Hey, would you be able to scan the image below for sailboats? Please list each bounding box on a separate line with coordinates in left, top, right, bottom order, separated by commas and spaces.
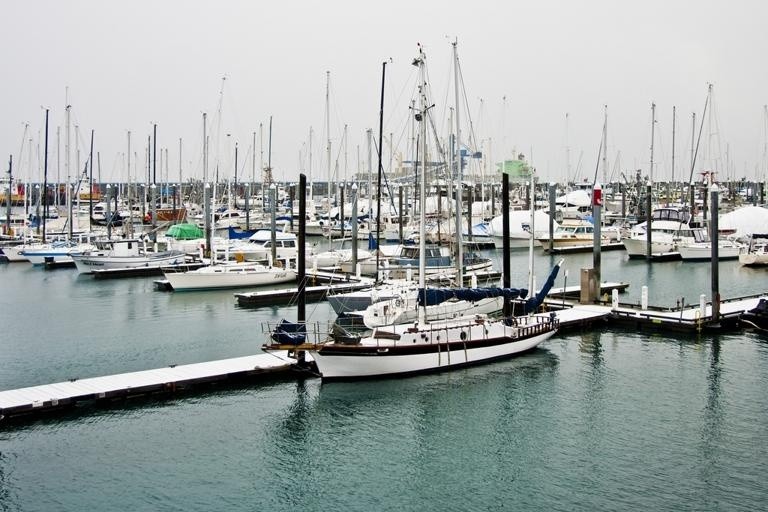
474, 83, 766, 272
0, 70, 393, 295
260, 35, 562, 398
